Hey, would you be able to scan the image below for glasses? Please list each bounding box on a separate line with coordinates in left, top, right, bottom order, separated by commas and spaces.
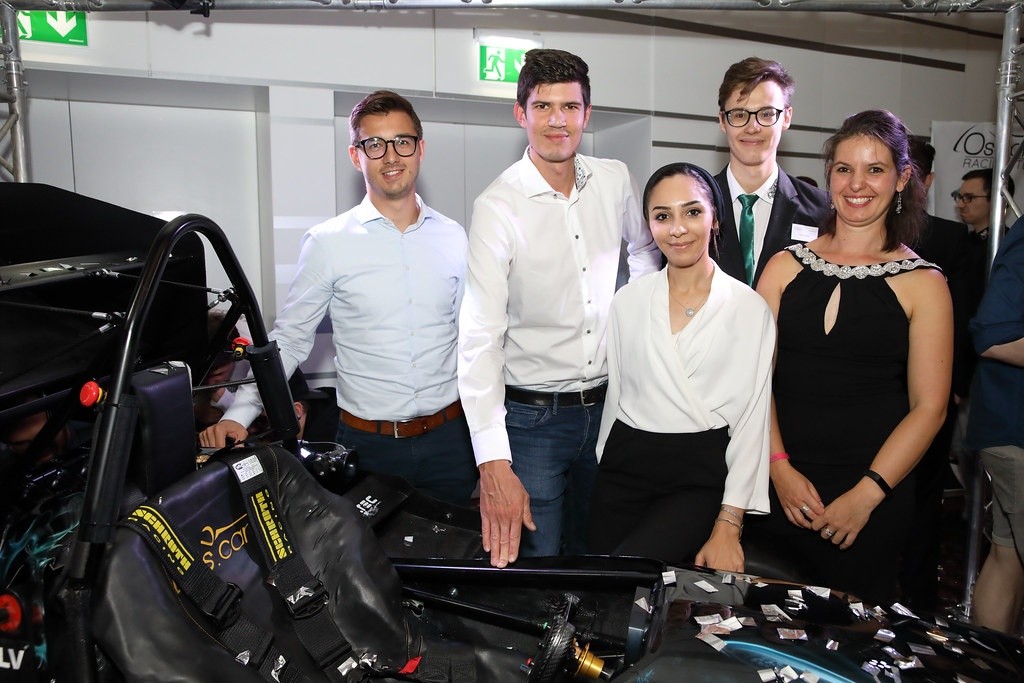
723, 107, 786, 128
951, 188, 989, 204
355, 135, 419, 159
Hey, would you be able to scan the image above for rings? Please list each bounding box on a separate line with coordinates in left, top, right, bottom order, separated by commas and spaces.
823, 526, 833, 537
801, 505, 809, 514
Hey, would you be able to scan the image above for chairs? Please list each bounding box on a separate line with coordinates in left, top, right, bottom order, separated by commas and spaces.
95, 448, 533, 683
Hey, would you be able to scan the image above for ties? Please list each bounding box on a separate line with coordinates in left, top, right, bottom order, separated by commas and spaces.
737, 194, 760, 287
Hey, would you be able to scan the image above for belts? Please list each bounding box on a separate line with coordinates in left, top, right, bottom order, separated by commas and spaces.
339, 399, 463, 439
505, 380, 608, 407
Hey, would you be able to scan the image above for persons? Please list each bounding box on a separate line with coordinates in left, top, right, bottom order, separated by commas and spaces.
190, 310, 338, 453
197, 48, 1024, 682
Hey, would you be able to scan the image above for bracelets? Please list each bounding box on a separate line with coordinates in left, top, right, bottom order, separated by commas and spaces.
770, 452, 790, 463
720, 506, 742, 522
714, 517, 743, 538
863, 469, 893, 497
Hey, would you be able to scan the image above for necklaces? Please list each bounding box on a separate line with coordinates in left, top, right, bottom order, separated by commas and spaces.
670, 293, 711, 317
